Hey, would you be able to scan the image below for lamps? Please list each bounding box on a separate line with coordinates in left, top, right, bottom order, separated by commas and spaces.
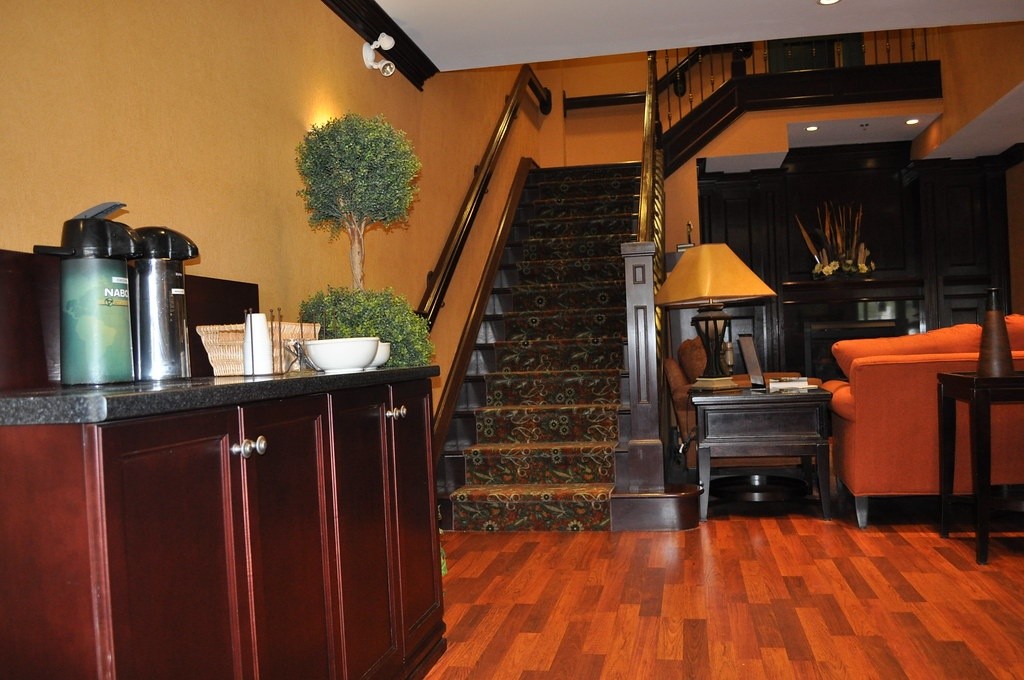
362, 32, 396, 77
656, 243, 777, 394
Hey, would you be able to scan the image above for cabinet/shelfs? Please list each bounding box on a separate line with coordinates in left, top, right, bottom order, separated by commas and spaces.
0, 364, 449, 680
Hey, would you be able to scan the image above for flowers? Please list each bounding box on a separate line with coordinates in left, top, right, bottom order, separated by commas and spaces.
812, 243, 876, 276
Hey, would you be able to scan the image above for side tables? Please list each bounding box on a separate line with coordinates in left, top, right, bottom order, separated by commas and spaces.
687, 387, 833, 521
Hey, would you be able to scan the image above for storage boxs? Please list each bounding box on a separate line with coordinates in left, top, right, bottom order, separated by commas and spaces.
196, 322, 321, 376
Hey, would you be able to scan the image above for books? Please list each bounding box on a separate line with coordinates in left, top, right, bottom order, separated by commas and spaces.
769, 377, 818, 393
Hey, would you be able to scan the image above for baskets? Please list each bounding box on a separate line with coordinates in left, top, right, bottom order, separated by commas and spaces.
196, 320, 322, 376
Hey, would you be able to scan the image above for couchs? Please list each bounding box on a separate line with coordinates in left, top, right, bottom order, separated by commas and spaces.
665, 336, 822, 496
820, 313, 1024, 529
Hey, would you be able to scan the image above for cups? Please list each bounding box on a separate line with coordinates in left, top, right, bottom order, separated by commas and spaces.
242, 312, 273, 375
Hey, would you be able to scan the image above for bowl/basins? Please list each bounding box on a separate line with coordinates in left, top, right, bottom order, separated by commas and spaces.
304, 337, 380, 376
364, 342, 390, 371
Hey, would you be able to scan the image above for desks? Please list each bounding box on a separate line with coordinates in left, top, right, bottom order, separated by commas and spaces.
930, 370, 1024, 561
